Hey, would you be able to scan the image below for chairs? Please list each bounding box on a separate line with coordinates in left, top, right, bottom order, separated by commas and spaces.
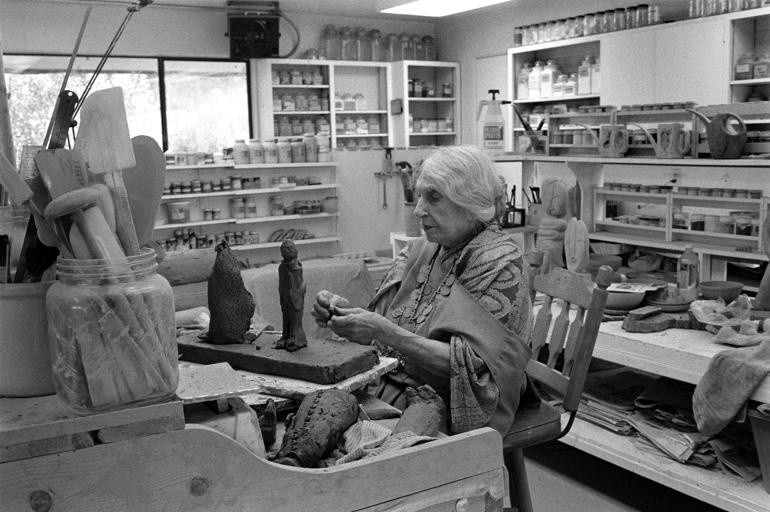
500, 248, 614, 511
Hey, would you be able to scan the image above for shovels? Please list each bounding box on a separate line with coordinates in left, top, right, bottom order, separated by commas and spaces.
35, 148, 89, 200
80, 87, 136, 258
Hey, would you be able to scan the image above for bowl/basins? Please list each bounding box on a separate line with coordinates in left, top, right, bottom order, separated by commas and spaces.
647, 288, 695, 313
701, 279, 743, 302
627, 277, 677, 292
594, 281, 645, 309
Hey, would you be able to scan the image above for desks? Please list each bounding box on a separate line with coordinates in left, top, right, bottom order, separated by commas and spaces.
0, 396, 506, 512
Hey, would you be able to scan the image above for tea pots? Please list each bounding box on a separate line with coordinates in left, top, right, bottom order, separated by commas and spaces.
629, 122, 689, 159
577, 121, 630, 158
685, 106, 747, 159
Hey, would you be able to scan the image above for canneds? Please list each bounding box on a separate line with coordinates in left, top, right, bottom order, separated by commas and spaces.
157, 238, 177, 252
198, 231, 260, 248
407, 76, 453, 98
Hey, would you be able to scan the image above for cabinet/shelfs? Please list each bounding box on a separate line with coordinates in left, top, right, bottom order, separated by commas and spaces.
153, 161, 340, 263
251, 57, 462, 151
504, 7, 770, 286
492, 100, 770, 511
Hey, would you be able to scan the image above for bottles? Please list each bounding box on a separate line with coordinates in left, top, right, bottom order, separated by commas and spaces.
386, 32, 401, 62
222, 178, 231, 190
231, 198, 245, 218
517, 63, 528, 99
593, 2, 656, 34
231, 175, 241, 189
540, 59, 561, 99
274, 116, 329, 136
555, 14, 594, 40
172, 181, 181, 193
410, 110, 453, 133
211, 180, 221, 191
291, 137, 306, 163
357, 25, 372, 61
232, 139, 250, 165
317, 135, 331, 163
522, 20, 555, 44
277, 137, 293, 163
590, 54, 601, 93
246, 197, 257, 218
182, 181, 191, 193
46, 247, 180, 413
272, 68, 324, 84
410, 33, 426, 60
370, 28, 385, 62
273, 91, 329, 110
249, 138, 265, 164
513, 26, 522, 46
577, 55, 592, 95
163, 183, 171, 194
734, 50, 754, 81
202, 180, 212, 192
687, 0, 769, 18
754, 47, 770, 80
302, 133, 320, 162
319, 24, 340, 60
193, 179, 201, 192
565, 72, 577, 97
335, 92, 367, 110
528, 61, 542, 99
398, 32, 414, 60
335, 115, 381, 135
175, 229, 197, 249
262, 138, 279, 164
553, 74, 567, 98
423, 35, 440, 61
271, 195, 284, 216
341, 25, 356, 60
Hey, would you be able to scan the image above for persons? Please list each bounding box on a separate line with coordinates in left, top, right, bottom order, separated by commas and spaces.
311, 144, 544, 439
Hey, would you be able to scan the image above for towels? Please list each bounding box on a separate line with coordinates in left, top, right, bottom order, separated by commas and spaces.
692, 327, 770, 437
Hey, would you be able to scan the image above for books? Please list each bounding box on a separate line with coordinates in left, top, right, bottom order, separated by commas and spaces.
530, 361, 761, 484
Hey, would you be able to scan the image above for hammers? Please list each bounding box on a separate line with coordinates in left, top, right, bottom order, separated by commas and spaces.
43, 187, 108, 258
